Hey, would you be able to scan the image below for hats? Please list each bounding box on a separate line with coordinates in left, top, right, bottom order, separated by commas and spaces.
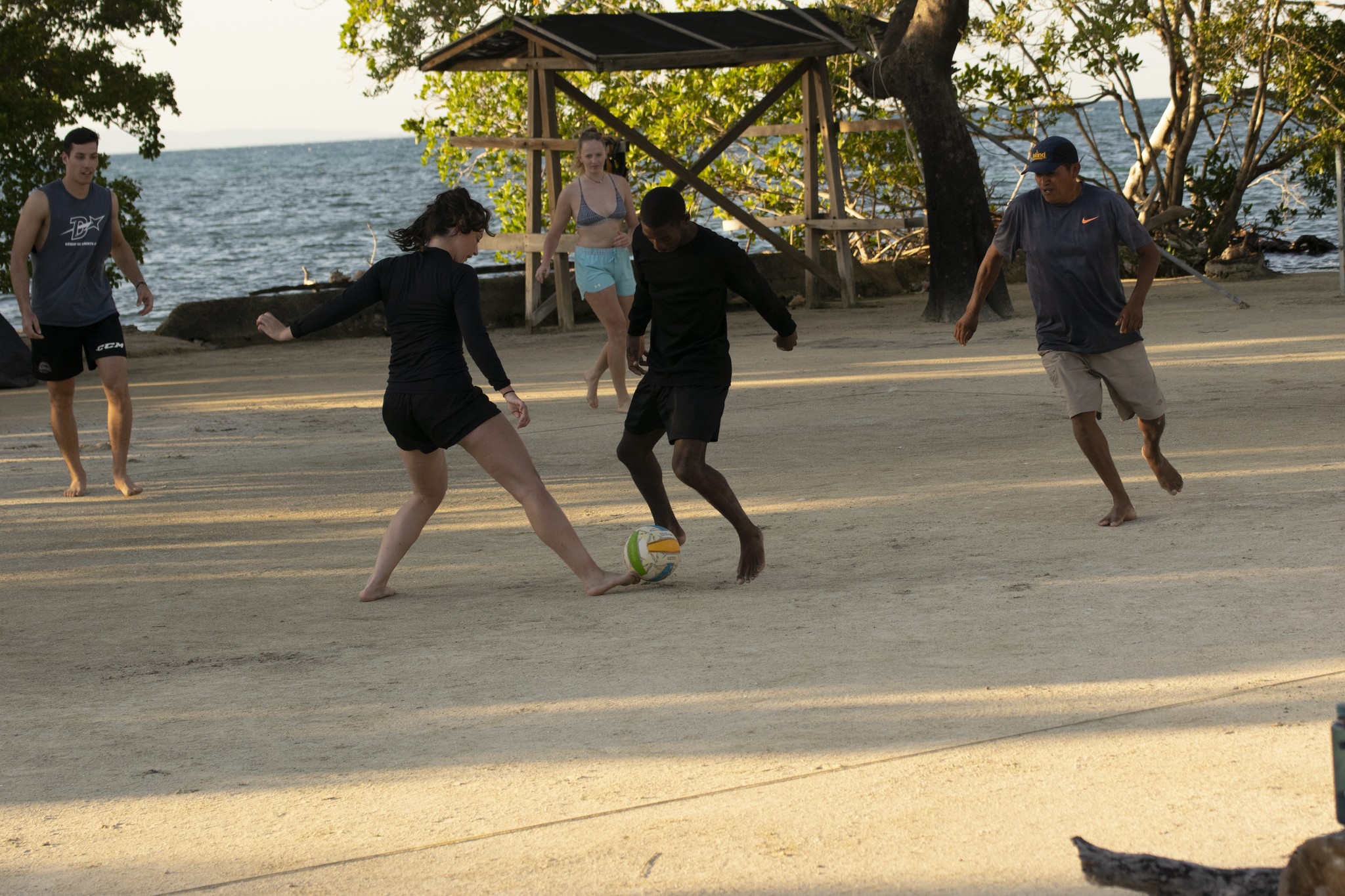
1020, 136, 1079, 175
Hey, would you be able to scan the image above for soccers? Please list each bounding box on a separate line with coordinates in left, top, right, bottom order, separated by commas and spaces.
623, 525, 681, 582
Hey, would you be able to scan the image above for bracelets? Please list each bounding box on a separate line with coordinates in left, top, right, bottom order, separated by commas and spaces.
503, 390, 515, 398
135, 282, 147, 291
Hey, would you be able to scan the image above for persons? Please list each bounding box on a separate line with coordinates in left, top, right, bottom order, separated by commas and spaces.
535, 128, 640, 413
10, 128, 153, 498
951, 136, 1186, 526
618, 187, 798, 584
256, 183, 641, 601
